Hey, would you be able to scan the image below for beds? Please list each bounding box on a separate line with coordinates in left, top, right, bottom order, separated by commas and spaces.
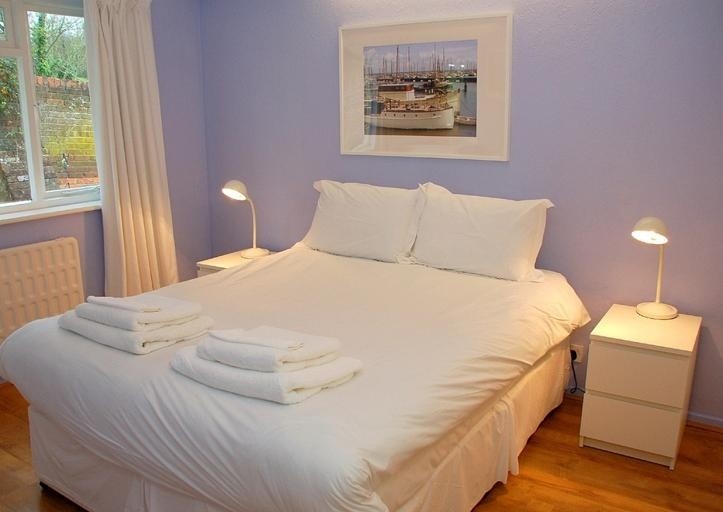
28, 243, 572, 511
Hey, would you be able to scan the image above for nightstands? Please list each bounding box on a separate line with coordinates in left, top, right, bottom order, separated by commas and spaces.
197, 251, 276, 278
578, 304, 702, 470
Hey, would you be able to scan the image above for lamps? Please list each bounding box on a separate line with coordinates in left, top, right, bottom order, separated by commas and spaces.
631, 217, 678, 319
221, 180, 269, 258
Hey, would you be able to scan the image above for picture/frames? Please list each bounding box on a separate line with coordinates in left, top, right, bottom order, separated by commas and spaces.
338, 11, 512, 163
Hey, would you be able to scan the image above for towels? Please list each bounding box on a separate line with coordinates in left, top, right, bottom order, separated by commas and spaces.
197, 326, 336, 372
171, 344, 360, 404
57, 310, 212, 355
76, 295, 201, 331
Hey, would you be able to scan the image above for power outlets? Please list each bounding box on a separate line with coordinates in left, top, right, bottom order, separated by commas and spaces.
570, 344, 582, 363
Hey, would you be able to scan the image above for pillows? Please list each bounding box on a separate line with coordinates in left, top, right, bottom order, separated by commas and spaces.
408, 181, 554, 282
300, 180, 425, 264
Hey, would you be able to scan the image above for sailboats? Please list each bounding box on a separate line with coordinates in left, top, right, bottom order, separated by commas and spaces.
364, 45, 476, 130
